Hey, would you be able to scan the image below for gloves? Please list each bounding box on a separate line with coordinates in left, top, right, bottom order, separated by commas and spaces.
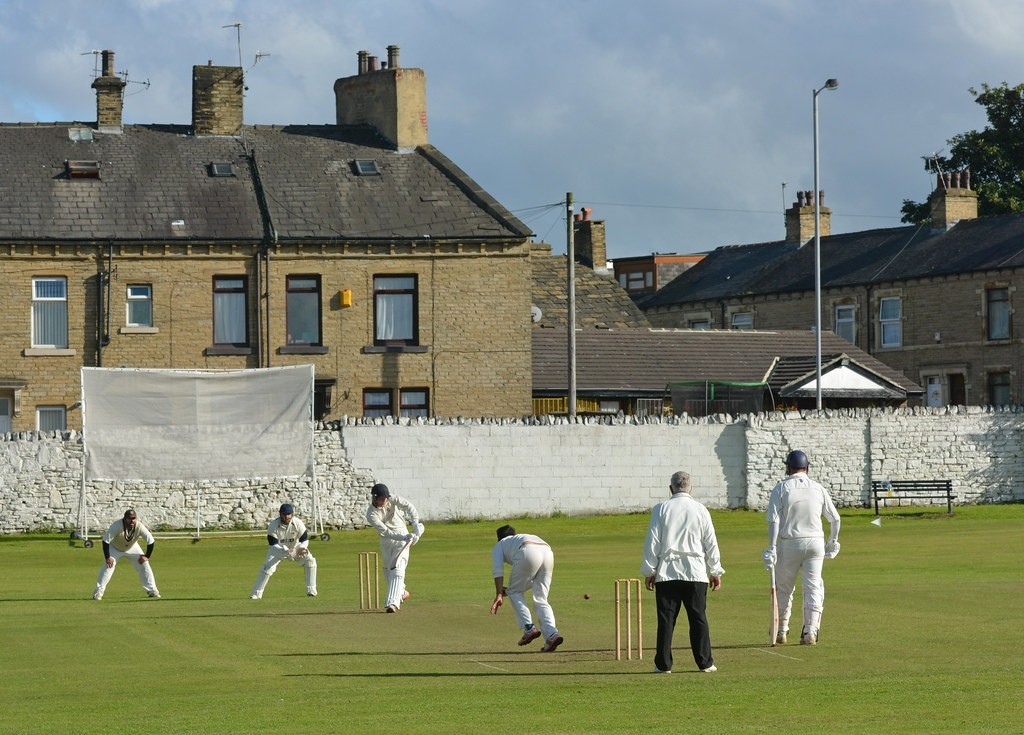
825, 542, 840, 559
411, 521, 425, 538
404, 533, 420, 545
760, 547, 777, 571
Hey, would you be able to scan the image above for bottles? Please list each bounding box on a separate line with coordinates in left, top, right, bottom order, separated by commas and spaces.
888, 482, 892, 496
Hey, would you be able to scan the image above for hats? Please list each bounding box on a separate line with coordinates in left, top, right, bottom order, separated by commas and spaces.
371, 484, 391, 497
281, 504, 293, 514
124, 510, 137, 518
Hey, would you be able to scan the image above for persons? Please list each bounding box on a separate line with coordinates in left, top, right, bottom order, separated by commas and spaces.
490, 525, 563, 653
644, 471, 725, 673
93, 510, 162, 601
367, 484, 421, 614
764, 451, 841, 645
249, 504, 318, 600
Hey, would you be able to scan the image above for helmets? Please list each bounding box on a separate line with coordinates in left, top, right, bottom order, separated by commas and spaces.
785, 450, 810, 476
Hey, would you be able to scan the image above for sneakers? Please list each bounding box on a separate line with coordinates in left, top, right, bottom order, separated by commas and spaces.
518, 624, 540, 646
540, 633, 564, 651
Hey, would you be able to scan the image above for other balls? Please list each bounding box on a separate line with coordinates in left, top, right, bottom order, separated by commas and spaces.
584, 593, 590, 600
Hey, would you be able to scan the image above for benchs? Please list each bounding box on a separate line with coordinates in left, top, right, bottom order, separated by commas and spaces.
870, 475, 958, 516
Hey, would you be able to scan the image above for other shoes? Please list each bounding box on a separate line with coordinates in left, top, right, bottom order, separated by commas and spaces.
250, 593, 258, 599
654, 666, 672, 674
400, 590, 410, 604
149, 593, 162, 597
700, 664, 717, 672
92, 595, 102, 600
776, 630, 787, 644
386, 606, 396, 613
800, 632, 817, 646
306, 588, 317, 596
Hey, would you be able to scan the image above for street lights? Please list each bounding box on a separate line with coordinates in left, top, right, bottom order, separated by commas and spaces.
811, 78, 838, 409
